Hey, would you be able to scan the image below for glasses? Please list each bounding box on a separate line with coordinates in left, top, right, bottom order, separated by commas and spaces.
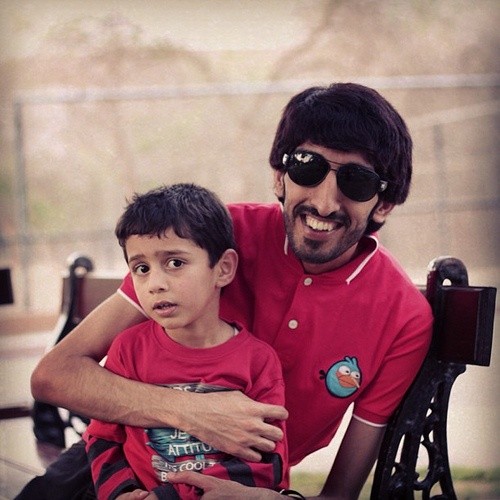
282, 150, 388, 202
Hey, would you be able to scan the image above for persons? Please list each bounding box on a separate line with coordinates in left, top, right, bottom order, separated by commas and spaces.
12, 82, 434, 499
82, 183, 291, 499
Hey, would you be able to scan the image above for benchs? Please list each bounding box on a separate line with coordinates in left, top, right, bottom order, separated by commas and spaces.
0, 256, 497, 500
